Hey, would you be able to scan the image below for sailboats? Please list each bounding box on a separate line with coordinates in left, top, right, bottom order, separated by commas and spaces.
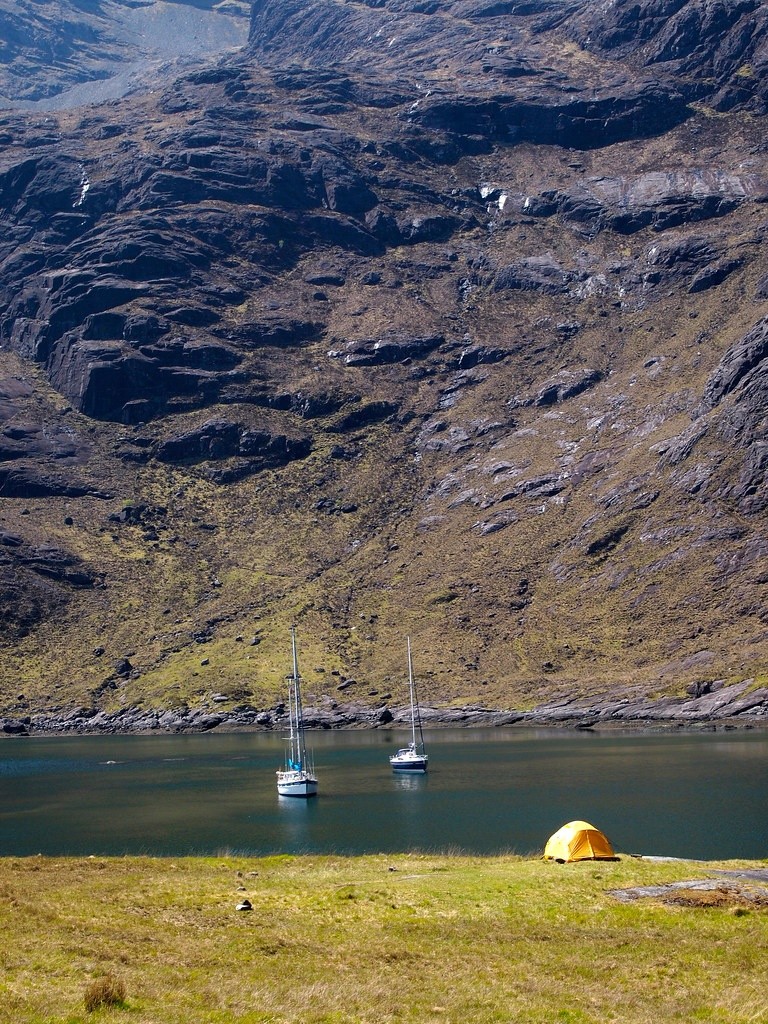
388, 635, 429, 774
274, 626, 319, 798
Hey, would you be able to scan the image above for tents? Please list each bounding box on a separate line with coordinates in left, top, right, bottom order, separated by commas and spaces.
542, 820, 621, 864
